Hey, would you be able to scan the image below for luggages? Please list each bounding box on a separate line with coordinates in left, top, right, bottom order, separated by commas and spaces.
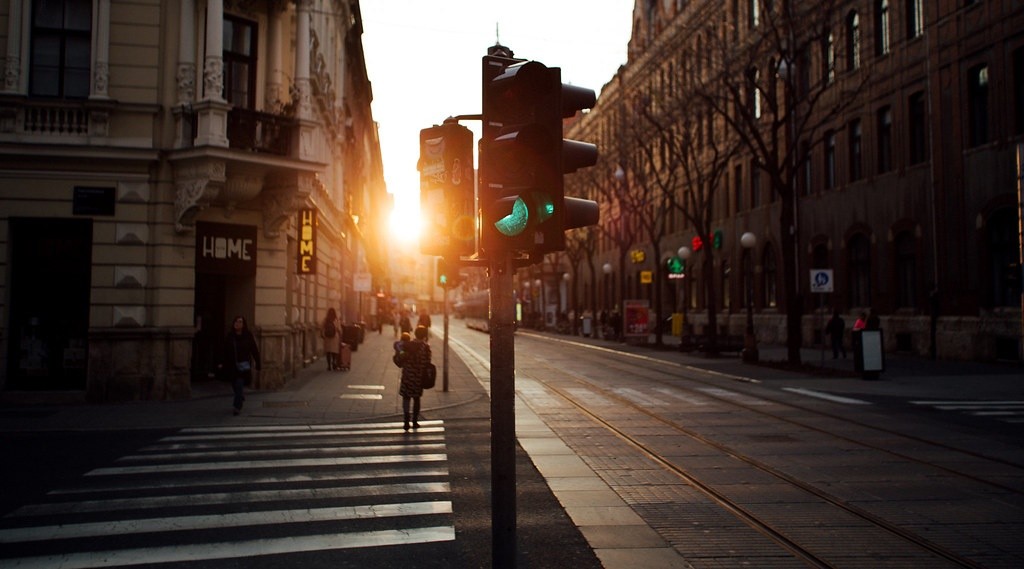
335, 343, 352, 371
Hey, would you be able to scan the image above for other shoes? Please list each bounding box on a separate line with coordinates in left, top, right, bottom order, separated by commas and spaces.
327, 367, 331, 371
333, 364, 336, 369
233, 408, 241, 416
403, 425, 410, 432
412, 423, 421, 429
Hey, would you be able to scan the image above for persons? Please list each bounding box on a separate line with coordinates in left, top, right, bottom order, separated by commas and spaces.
854, 312, 866, 330
866, 306, 881, 331
393, 332, 411, 355
824, 308, 848, 361
417, 308, 431, 342
398, 313, 412, 338
393, 326, 432, 431
320, 308, 342, 371
216, 315, 261, 417
376, 308, 384, 335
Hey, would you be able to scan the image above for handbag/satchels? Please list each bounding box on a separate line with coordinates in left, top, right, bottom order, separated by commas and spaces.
420, 363, 436, 389
235, 361, 251, 378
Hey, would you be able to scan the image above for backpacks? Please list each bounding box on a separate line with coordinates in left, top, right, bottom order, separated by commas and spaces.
324, 318, 336, 338
